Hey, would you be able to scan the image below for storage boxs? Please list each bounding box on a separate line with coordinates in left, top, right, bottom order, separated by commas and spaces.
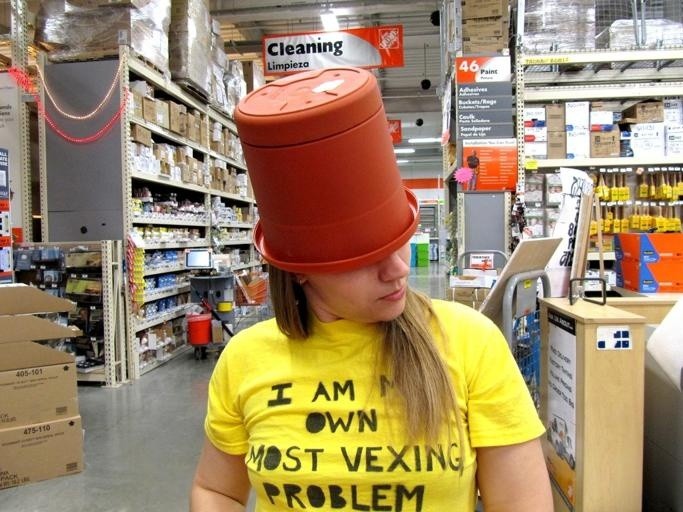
1, 416, 87, 490
0, 362, 80, 430
49, 1, 251, 189
409, 240, 430, 268
439, 2, 683, 162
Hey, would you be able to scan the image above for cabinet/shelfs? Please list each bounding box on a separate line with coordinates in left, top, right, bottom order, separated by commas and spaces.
36, 50, 270, 390
440, 52, 682, 335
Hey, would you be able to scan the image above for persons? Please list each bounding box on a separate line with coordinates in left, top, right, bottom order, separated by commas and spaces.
466, 149, 480, 191
188, 239, 555, 512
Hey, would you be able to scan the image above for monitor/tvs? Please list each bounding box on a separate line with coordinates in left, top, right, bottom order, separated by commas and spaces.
186, 248, 213, 270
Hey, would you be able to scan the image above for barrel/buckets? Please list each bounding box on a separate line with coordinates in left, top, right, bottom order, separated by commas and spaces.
417, 243, 428, 267
409, 244, 417, 267
188, 314, 212, 344
235, 69, 421, 273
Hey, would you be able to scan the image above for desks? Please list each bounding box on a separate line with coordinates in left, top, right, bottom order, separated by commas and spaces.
534, 292, 683, 511
